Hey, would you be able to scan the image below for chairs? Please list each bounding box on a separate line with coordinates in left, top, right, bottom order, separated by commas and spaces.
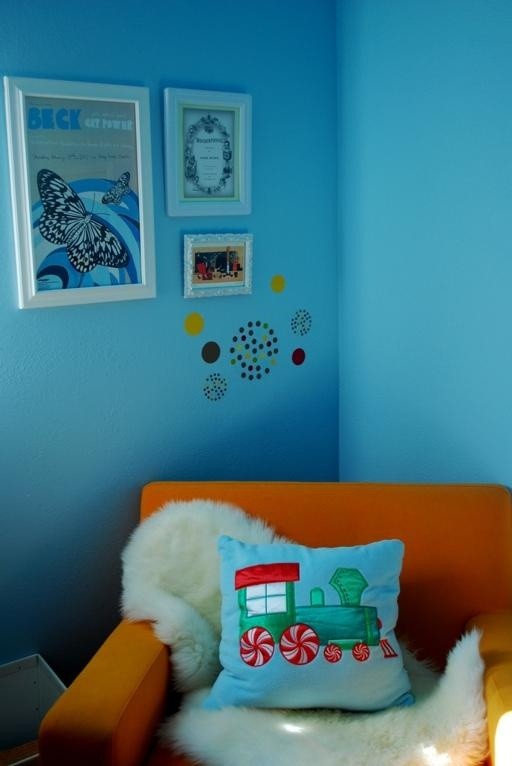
39, 480, 512, 766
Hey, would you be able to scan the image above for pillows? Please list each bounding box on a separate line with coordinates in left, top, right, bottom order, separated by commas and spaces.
196, 532, 416, 715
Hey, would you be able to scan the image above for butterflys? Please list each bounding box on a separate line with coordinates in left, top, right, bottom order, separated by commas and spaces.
101, 171, 131, 206
35, 167, 129, 274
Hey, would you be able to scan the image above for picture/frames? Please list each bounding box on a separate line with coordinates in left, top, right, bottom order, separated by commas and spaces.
179, 228, 254, 304
3, 73, 164, 310
162, 85, 252, 219
